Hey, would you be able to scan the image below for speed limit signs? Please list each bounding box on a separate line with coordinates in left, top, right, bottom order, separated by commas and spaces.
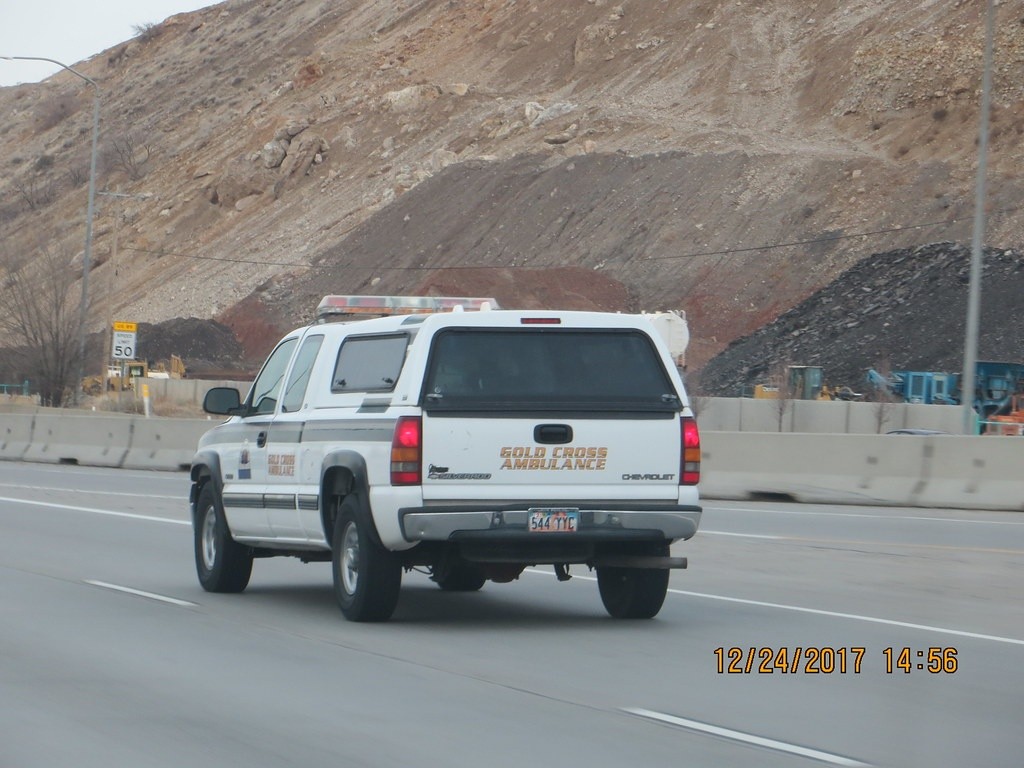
112, 330, 137, 360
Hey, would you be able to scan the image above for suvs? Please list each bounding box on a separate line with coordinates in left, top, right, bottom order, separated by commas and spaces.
189, 294, 703, 622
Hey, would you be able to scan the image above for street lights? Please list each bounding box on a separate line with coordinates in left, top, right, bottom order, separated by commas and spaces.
0, 56, 102, 405
